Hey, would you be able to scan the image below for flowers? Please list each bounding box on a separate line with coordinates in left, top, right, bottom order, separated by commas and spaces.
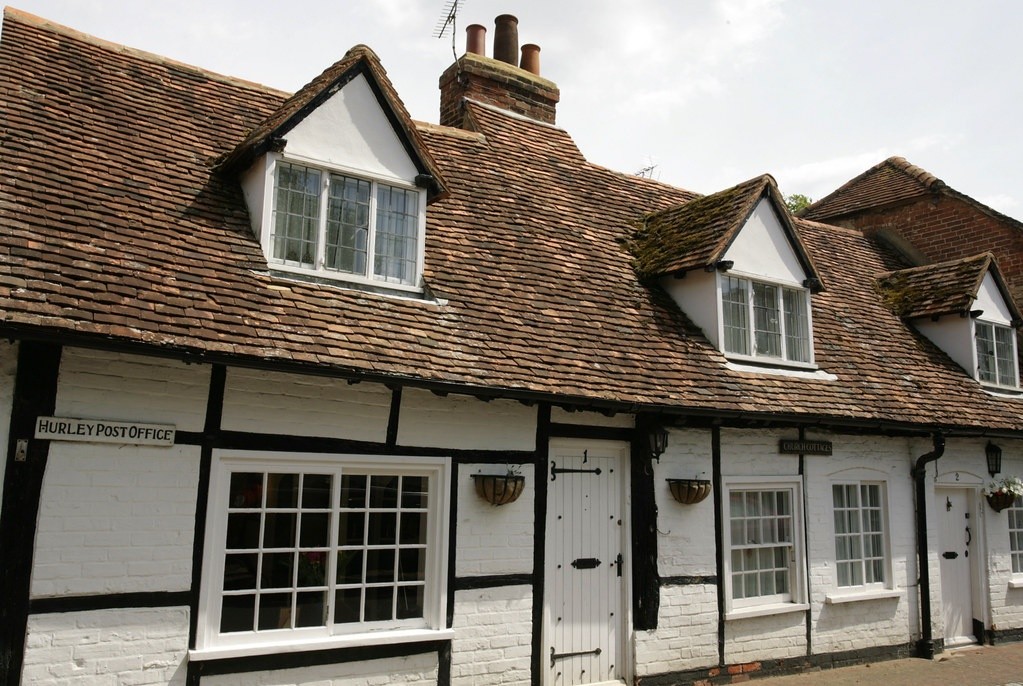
981, 476, 1022, 498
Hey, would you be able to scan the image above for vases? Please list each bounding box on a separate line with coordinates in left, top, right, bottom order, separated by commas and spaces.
987, 493, 1017, 513
